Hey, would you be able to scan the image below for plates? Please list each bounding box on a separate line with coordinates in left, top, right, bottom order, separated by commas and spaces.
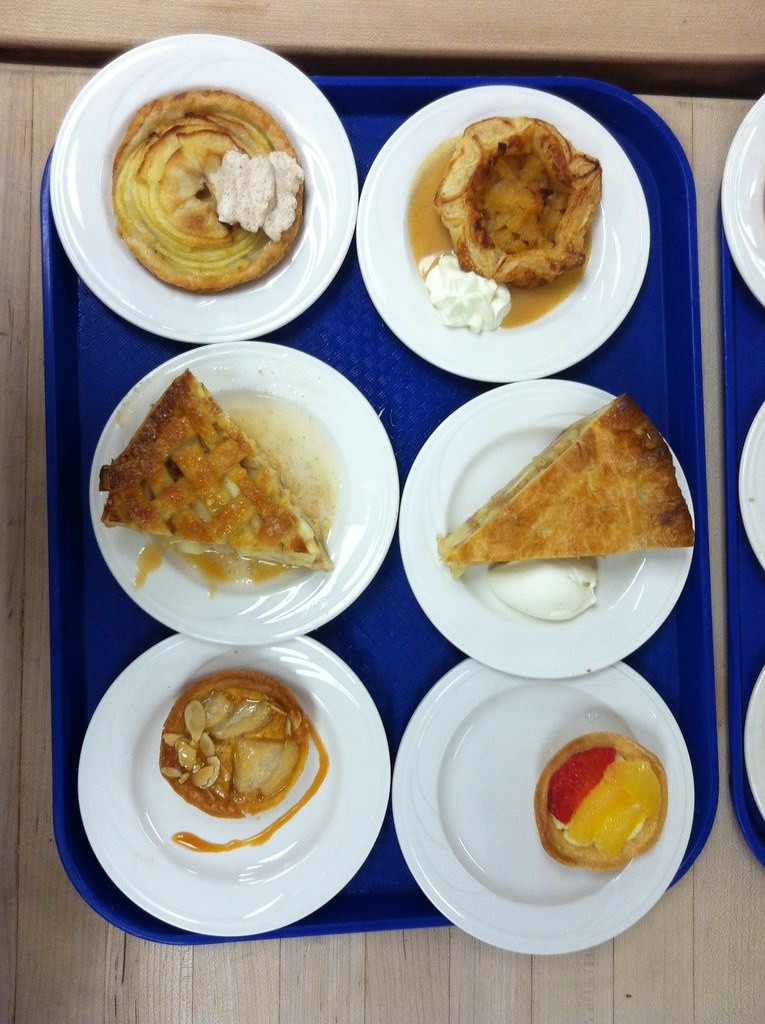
390, 656, 694, 956
399, 378, 695, 676
50, 32, 363, 343
743, 665, 765, 823
720, 93, 765, 309
738, 401, 765, 571
354, 83, 650, 380
87, 342, 399, 647
77, 633, 392, 936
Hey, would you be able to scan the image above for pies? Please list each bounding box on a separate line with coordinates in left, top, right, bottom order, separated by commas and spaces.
94, 370, 333, 571
437, 391, 694, 574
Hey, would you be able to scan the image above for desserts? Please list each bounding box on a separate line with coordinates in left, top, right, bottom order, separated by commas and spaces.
156, 669, 310, 818
432, 114, 603, 288
533, 730, 667, 871
111, 89, 305, 292
